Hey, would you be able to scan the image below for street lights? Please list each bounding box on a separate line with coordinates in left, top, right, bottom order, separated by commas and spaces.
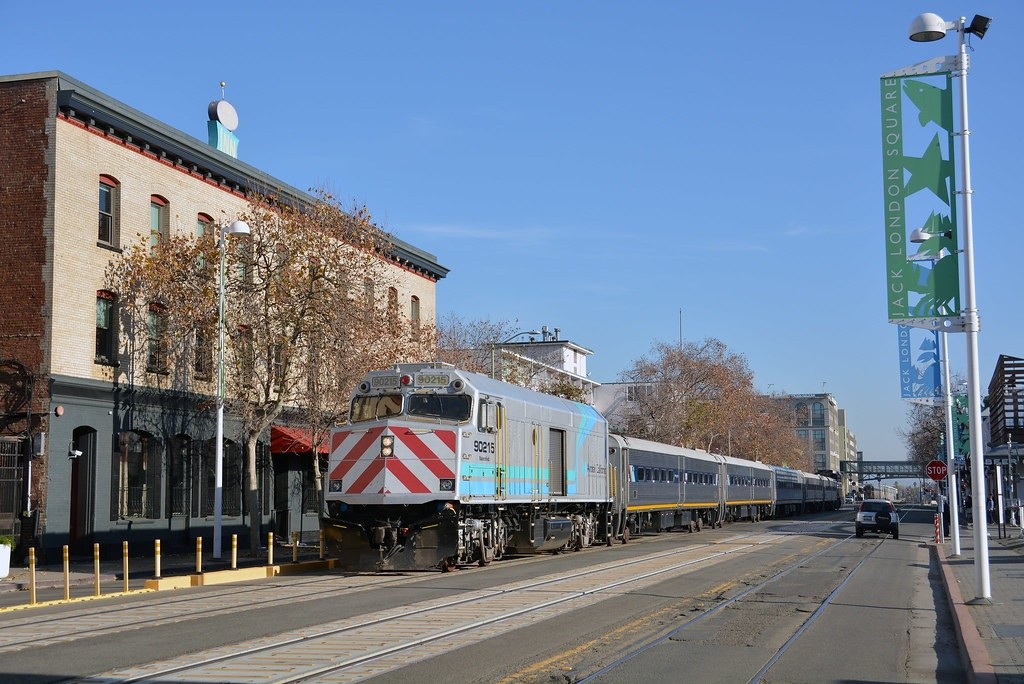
911, 228, 961, 557
212, 220, 250, 564
908, 11, 998, 607
491, 331, 541, 379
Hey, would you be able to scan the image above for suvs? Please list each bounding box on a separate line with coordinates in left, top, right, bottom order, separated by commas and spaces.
855, 498, 900, 540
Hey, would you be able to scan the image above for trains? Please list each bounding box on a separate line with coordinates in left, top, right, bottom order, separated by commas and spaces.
313, 361, 844, 573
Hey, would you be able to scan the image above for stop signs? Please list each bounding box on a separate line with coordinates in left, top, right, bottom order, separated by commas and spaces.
926, 460, 948, 480
